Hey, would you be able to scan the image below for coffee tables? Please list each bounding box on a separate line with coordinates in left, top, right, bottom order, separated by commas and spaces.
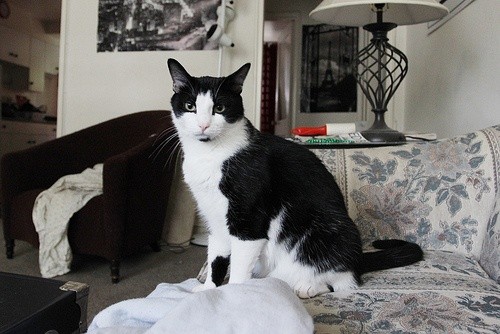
0, 270, 89, 334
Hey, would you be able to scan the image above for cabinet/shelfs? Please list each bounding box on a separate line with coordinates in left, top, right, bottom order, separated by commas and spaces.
1, 121, 57, 156
0, 21, 59, 121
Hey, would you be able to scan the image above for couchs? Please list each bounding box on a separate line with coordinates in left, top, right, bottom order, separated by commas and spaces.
1, 111, 181, 284
83, 125, 499, 334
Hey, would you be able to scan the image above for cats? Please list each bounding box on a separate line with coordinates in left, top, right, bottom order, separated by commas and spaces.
167, 57, 423, 299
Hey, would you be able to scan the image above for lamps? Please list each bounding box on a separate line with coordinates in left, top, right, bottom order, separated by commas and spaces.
309, 0, 450, 143
